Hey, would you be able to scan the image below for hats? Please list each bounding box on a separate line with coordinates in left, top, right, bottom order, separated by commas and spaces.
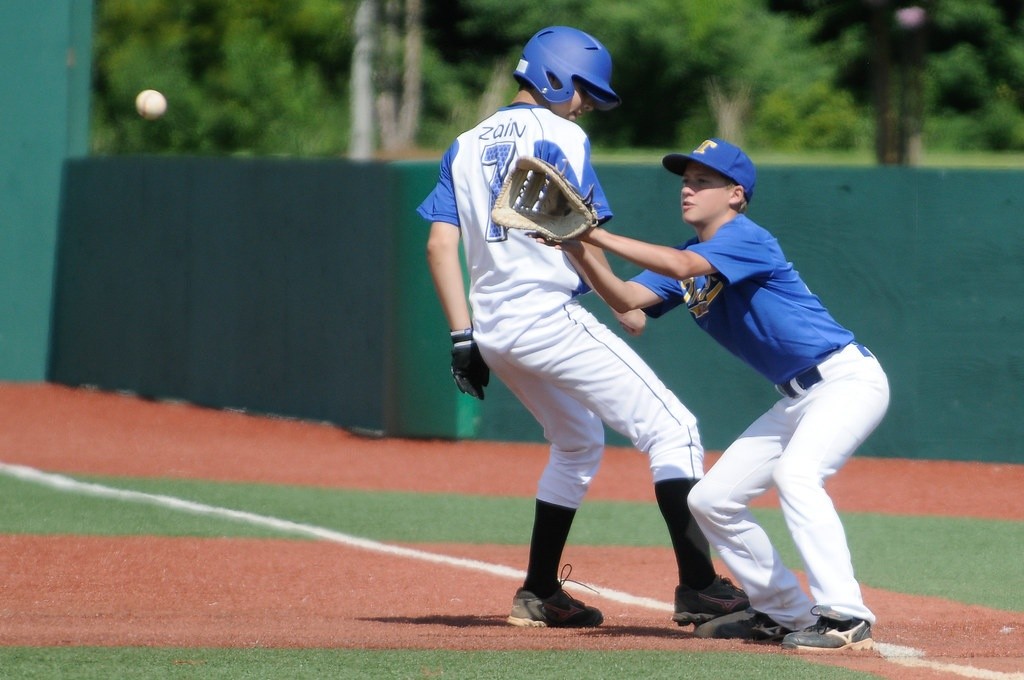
661, 137, 759, 205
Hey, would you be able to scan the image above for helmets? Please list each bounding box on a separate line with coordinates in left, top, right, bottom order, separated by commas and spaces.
513, 26, 622, 113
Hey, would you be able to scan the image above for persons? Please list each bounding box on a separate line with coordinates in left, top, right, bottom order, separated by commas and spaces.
416, 25, 752, 629
526, 137, 890, 653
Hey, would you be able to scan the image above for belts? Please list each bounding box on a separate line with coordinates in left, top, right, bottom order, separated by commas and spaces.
777, 347, 874, 396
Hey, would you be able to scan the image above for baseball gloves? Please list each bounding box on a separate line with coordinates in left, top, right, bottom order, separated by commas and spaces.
488, 155, 600, 245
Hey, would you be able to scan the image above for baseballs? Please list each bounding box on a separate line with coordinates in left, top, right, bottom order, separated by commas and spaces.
134, 88, 168, 120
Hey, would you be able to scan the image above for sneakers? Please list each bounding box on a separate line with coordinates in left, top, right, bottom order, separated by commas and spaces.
674, 576, 752, 624
783, 604, 874, 654
507, 584, 605, 628
718, 613, 790, 643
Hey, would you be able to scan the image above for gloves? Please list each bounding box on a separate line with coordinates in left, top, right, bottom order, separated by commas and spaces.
449, 330, 494, 401
